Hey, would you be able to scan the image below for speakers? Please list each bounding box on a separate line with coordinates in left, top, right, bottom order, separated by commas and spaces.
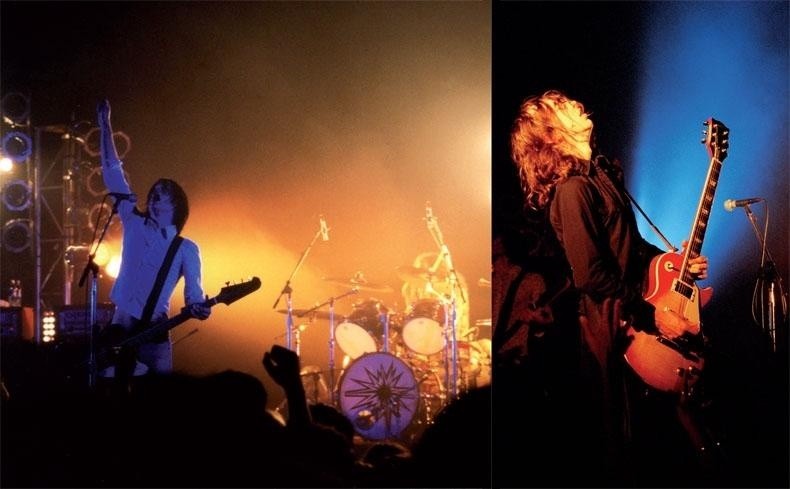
38, 131, 66, 310
74, 115, 113, 244
1, 88, 35, 307
109, 128, 131, 188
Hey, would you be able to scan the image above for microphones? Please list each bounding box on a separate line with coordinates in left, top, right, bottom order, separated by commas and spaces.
106, 191, 138, 204
316, 214, 329, 241
423, 200, 433, 222
724, 197, 765, 211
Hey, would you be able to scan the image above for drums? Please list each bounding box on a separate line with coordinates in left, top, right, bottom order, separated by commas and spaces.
433, 340, 482, 378
401, 299, 448, 356
339, 352, 420, 441
334, 299, 392, 360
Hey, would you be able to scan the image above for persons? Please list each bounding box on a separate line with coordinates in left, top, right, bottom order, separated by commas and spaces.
510, 90, 707, 447
95, 99, 209, 384
3, 340, 410, 485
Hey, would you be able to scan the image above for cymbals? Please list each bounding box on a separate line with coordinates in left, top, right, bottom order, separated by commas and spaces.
277, 308, 344, 322
323, 278, 395, 296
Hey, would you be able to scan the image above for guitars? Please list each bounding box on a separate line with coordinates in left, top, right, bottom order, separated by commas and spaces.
622, 117, 730, 398
78, 277, 260, 388
